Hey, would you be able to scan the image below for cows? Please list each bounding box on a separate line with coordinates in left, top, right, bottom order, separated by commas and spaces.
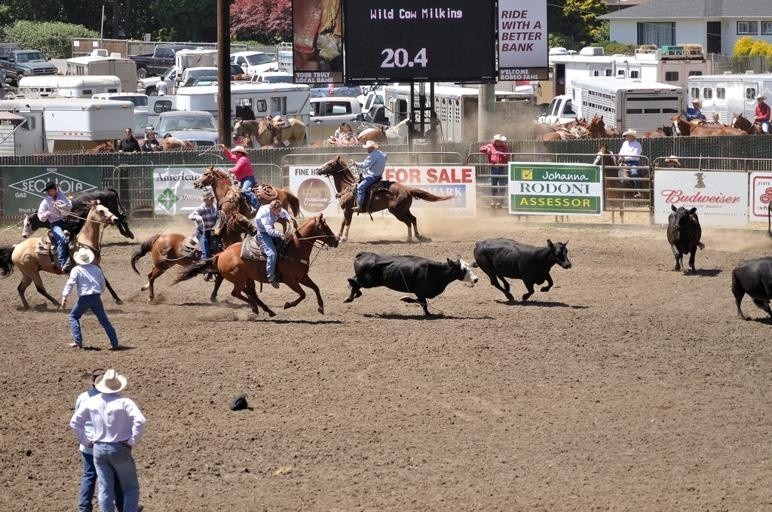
470, 237, 570, 301
22, 188, 135, 240
343, 251, 478, 317
730, 256, 772, 321
666, 204, 706, 274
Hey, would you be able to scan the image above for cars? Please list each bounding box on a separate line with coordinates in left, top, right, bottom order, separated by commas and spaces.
309, 96, 363, 143
179, 67, 219, 89
149, 109, 220, 150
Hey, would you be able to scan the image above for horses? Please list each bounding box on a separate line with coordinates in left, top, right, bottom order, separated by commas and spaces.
0, 200, 124, 310
309, 117, 415, 149
227, 116, 308, 148
592, 145, 652, 223
131, 210, 258, 302
170, 213, 339, 318
497, 111, 772, 140
86, 136, 198, 153
316, 155, 455, 242
193, 165, 305, 234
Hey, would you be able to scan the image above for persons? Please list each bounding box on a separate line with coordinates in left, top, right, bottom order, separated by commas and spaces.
351, 140, 388, 214
617, 127, 645, 199
118, 129, 141, 152
253, 197, 294, 291
663, 153, 683, 168
156, 75, 170, 96
59, 247, 120, 352
69, 369, 149, 511
37, 181, 73, 274
142, 126, 164, 151
217, 143, 259, 210
339, 123, 354, 139
752, 94, 771, 136
479, 134, 512, 212
679, 99, 707, 124
73, 370, 105, 511
710, 111, 724, 126
187, 191, 223, 283
334, 122, 346, 139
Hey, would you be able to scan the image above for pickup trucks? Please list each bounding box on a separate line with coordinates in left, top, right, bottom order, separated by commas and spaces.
358, 90, 386, 118
538, 95, 577, 127
0, 49, 59, 87
128, 44, 195, 80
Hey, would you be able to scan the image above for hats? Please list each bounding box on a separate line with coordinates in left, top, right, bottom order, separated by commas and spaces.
755, 95, 767, 99
622, 128, 637, 137
490, 134, 507, 144
41, 181, 59, 192
72, 247, 95, 266
692, 99, 703, 108
94, 368, 128, 394
202, 191, 214, 201
362, 140, 379, 149
231, 145, 248, 155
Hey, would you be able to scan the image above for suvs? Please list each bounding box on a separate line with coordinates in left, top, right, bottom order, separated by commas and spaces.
137, 65, 178, 98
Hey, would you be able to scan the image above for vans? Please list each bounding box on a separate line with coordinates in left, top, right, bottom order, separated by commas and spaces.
91, 92, 150, 124
230, 51, 278, 77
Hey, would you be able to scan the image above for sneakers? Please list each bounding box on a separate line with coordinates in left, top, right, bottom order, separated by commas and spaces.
351, 205, 363, 212
62, 267, 70, 274
69, 342, 83, 349
108, 345, 119, 350
271, 279, 280, 289
634, 193, 642, 198
490, 202, 502, 209
